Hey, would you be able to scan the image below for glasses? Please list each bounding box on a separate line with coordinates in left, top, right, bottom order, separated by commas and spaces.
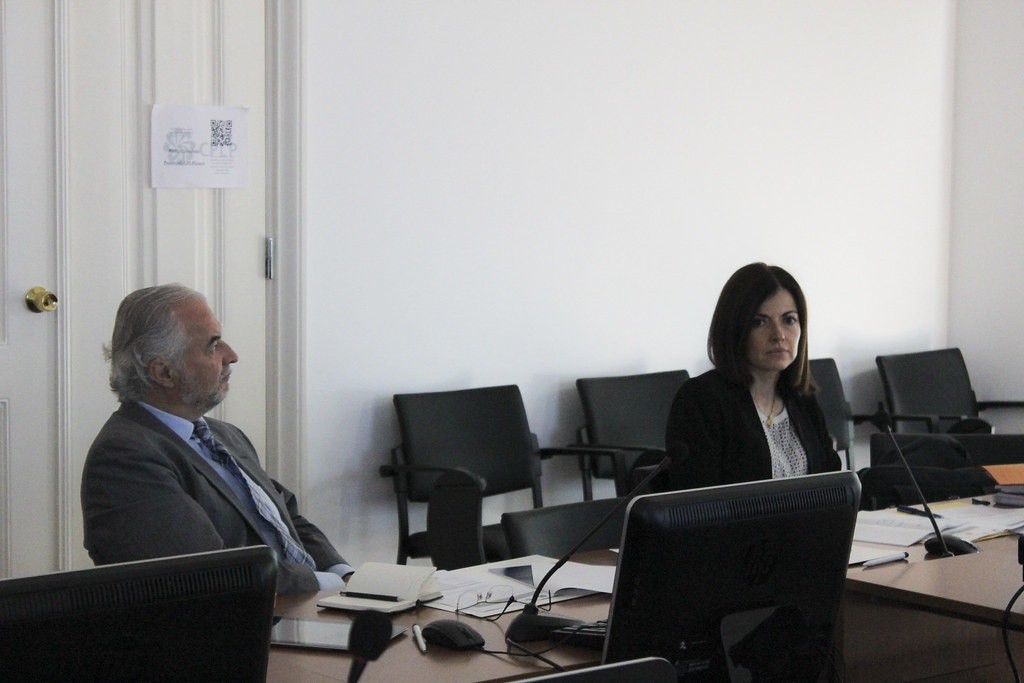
455, 583, 552, 622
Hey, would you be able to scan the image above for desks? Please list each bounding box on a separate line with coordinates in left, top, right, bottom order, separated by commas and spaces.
267, 543, 619, 683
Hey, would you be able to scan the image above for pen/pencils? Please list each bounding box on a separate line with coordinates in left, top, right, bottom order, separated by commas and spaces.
340, 592, 406, 602
411, 623, 427, 652
863, 552, 909, 568
897, 505, 943, 519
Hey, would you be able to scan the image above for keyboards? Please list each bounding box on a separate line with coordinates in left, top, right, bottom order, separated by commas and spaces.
548, 618, 608, 647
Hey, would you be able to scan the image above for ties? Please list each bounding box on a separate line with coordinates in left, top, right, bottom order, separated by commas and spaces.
190, 420, 318, 574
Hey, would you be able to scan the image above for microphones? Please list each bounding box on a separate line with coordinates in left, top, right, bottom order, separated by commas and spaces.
505, 441, 691, 644
345, 610, 394, 683
871, 410, 957, 557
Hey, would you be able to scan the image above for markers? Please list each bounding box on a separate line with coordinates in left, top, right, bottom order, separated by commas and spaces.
972, 498, 990, 506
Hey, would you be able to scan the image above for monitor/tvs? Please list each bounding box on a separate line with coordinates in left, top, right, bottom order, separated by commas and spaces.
601, 471, 863, 683
0, 544, 277, 683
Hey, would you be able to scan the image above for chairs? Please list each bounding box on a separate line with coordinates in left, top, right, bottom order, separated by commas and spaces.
502, 494, 625, 558
843, 490, 1024, 683
807, 360, 999, 511
379, 385, 544, 572
875, 348, 1024, 499
569, 370, 692, 500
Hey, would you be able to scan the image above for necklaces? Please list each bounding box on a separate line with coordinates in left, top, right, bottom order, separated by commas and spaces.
751, 385, 778, 427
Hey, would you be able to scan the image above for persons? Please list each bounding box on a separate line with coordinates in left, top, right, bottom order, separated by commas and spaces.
80, 284, 356, 595
663, 262, 842, 491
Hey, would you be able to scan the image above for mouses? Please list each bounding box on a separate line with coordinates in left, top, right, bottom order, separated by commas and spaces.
423, 621, 486, 649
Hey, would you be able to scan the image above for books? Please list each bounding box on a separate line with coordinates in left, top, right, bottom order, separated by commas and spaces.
317, 561, 443, 614
994, 484, 1024, 505
